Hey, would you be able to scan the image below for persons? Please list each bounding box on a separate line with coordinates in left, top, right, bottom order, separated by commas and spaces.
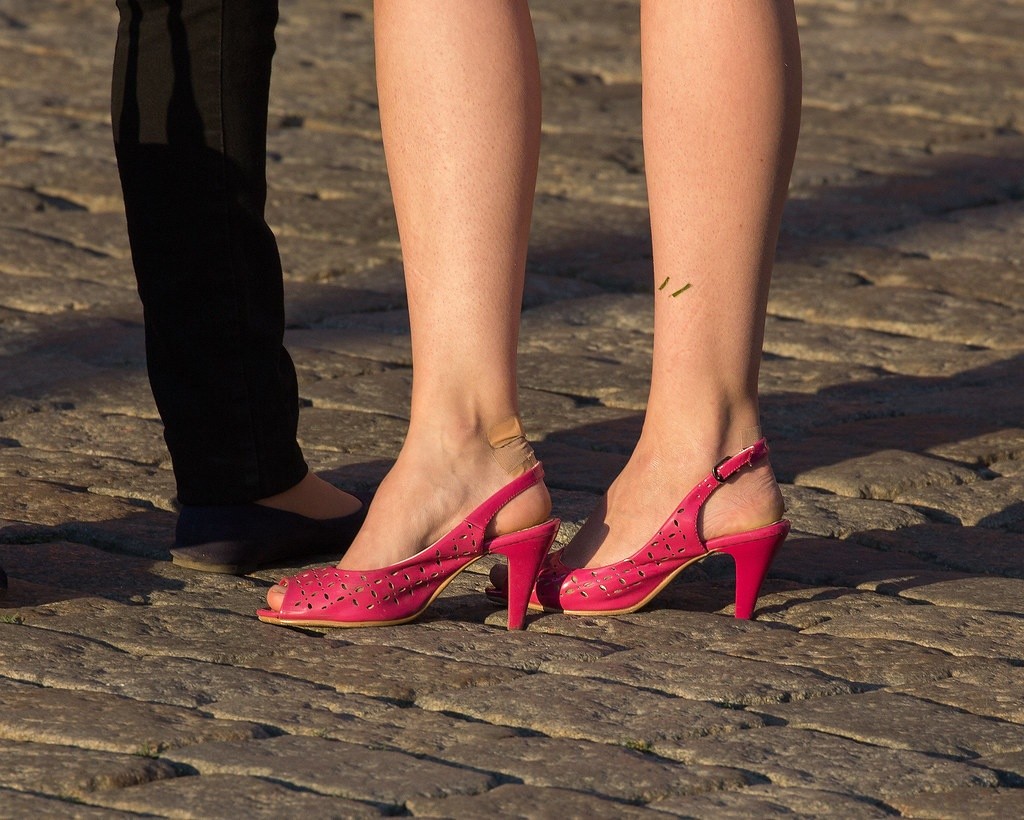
257, 0, 802, 626
111, 0, 374, 570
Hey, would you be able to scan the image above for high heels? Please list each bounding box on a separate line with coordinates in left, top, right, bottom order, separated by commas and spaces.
485, 437, 791, 621
255, 459, 560, 631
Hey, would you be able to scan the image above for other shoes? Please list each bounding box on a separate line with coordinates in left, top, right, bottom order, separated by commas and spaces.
169, 484, 376, 573
